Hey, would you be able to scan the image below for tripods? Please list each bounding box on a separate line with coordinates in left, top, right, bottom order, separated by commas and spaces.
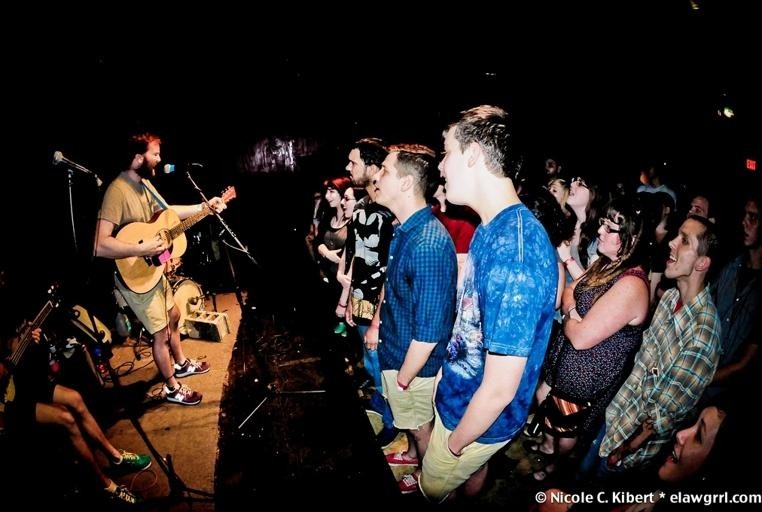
64, 179, 214, 511
217, 228, 327, 433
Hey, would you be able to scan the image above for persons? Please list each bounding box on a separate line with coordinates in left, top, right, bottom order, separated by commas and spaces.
0, 312, 151, 506
308, 106, 761, 512
93, 132, 228, 406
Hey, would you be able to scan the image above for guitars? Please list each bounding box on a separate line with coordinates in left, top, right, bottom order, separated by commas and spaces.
0, 284, 62, 431
110, 185, 236, 294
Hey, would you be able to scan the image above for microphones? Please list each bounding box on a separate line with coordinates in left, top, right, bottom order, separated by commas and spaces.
163, 162, 204, 176
53, 148, 99, 182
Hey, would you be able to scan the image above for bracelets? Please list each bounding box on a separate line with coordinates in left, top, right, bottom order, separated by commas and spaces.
201, 201, 205, 210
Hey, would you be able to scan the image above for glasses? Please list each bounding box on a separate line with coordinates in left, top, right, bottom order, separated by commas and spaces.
598, 218, 620, 234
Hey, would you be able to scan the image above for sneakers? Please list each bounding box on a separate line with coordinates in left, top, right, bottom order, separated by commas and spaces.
361, 397, 420, 495
104, 449, 152, 506
523, 439, 556, 458
163, 358, 211, 406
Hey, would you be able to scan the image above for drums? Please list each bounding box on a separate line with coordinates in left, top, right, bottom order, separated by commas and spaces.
169, 276, 204, 340
